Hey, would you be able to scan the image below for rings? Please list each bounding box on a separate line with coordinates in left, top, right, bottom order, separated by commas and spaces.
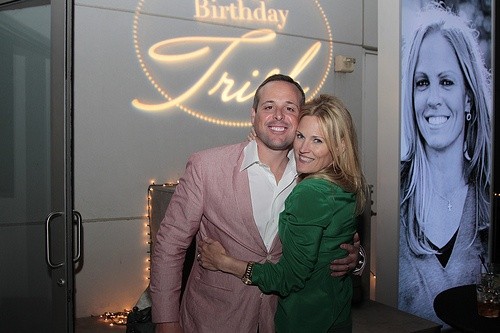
346, 264, 350, 272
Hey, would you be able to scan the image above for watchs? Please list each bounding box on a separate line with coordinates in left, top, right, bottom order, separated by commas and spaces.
240, 259, 256, 285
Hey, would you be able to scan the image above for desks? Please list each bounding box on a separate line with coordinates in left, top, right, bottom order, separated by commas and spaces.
433, 284, 500, 333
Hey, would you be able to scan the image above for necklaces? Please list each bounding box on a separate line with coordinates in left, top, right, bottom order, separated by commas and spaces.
432, 175, 464, 211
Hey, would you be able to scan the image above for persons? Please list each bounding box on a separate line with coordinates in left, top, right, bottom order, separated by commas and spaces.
198, 93, 370, 333
150, 74, 371, 333
399, 16, 494, 329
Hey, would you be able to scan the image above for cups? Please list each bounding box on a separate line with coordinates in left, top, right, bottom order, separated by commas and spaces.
475, 274, 500, 317
